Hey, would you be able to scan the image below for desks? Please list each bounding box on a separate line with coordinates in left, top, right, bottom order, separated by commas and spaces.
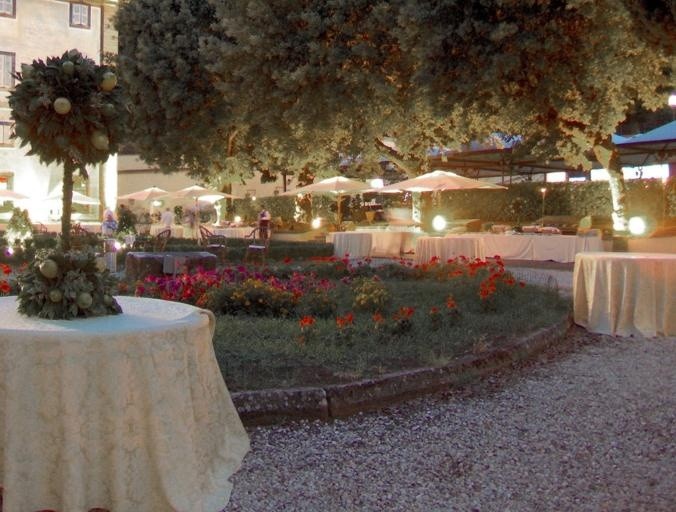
571, 252, 675, 338
412, 236, 483, 265
0, 296, 219, 510
325, 230, 410, 261
468, 231, 604, 263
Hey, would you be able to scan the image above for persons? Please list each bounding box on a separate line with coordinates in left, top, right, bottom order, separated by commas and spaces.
98, 211, 116, 234
160, 207, 173, 225
258, 204, 272, 239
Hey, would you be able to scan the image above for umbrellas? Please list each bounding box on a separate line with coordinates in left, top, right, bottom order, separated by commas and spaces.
277, 174, 404, 229
150, 184, 243, 237
0, 189, 31, 202
115, 186, 170, 201
376, 169, 510, 210
40, 188, 101, 209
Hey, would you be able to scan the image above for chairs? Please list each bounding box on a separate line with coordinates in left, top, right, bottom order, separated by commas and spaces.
131, 229, 171, 252
198, 223, 268, 266
